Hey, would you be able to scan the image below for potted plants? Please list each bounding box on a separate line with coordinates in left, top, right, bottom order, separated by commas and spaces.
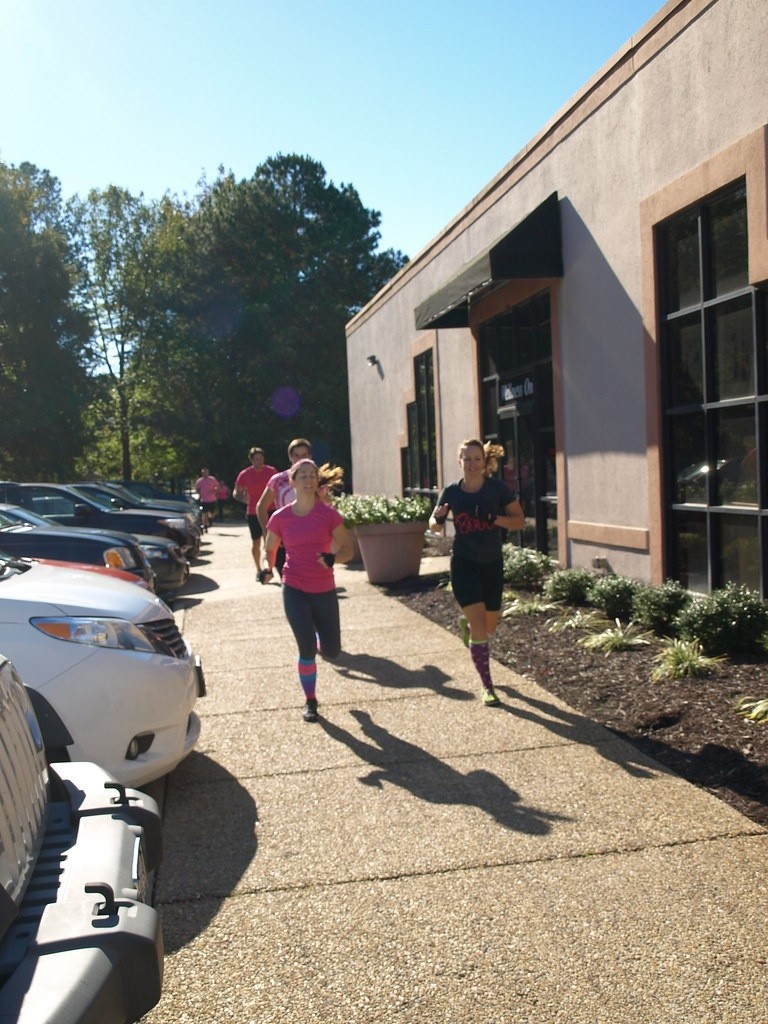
337, 495, 430, 586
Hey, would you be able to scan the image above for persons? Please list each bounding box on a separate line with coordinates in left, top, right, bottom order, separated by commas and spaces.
428, 438, 525, 707
217, 480, 232, 522
233, 447, 278, 582
195, 468, 219, 533
258, 458, 348, 721
257, 438, 329, 582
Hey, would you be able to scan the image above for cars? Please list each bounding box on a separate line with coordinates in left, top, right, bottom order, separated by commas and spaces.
23, 496, 189, 591
72, 483, 208, 534
0, 552, 207, 788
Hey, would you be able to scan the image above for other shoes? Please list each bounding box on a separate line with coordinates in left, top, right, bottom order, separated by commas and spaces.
256, 571, 263, 581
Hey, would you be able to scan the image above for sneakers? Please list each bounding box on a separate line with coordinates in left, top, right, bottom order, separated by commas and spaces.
482, 689, 499, 705
459, 616, 469, 647
303, 699, 319, 722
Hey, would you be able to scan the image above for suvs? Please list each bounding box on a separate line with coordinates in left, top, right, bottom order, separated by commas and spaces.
0, 504, 155, 594
0, 652, 167, 1024
0, 481, 202, 558
